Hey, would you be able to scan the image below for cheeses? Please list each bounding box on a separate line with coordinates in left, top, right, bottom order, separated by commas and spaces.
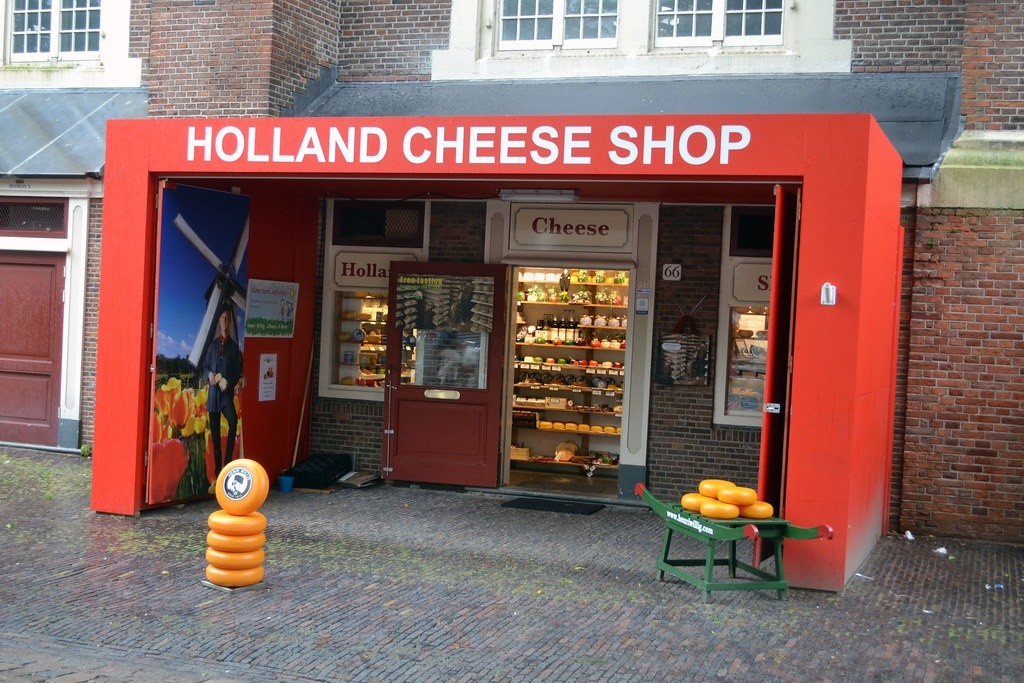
206, 458, 269, 586
681, 479, 773, 518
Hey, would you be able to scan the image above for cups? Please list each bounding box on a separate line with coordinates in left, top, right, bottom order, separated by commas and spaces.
278, 476, 294, 493
344, 351, 355, 364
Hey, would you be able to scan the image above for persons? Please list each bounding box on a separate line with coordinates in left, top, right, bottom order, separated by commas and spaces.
415, 278, 473, 330
402, 330, 416, 362
263, 368, 273, 379
204, 301, 243, 480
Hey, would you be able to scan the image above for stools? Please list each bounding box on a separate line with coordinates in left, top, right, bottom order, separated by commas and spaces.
634, 482, 834, 603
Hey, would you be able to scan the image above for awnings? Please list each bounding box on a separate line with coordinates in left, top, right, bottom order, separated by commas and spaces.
290, 71, 969, 187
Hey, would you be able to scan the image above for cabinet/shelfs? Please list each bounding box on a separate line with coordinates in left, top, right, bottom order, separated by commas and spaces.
510, 274, 628, 480
335, 293, 388, 391
728, 306, 770, 391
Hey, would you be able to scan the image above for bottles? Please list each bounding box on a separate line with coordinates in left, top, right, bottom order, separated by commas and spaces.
535, 314, 582, 342
